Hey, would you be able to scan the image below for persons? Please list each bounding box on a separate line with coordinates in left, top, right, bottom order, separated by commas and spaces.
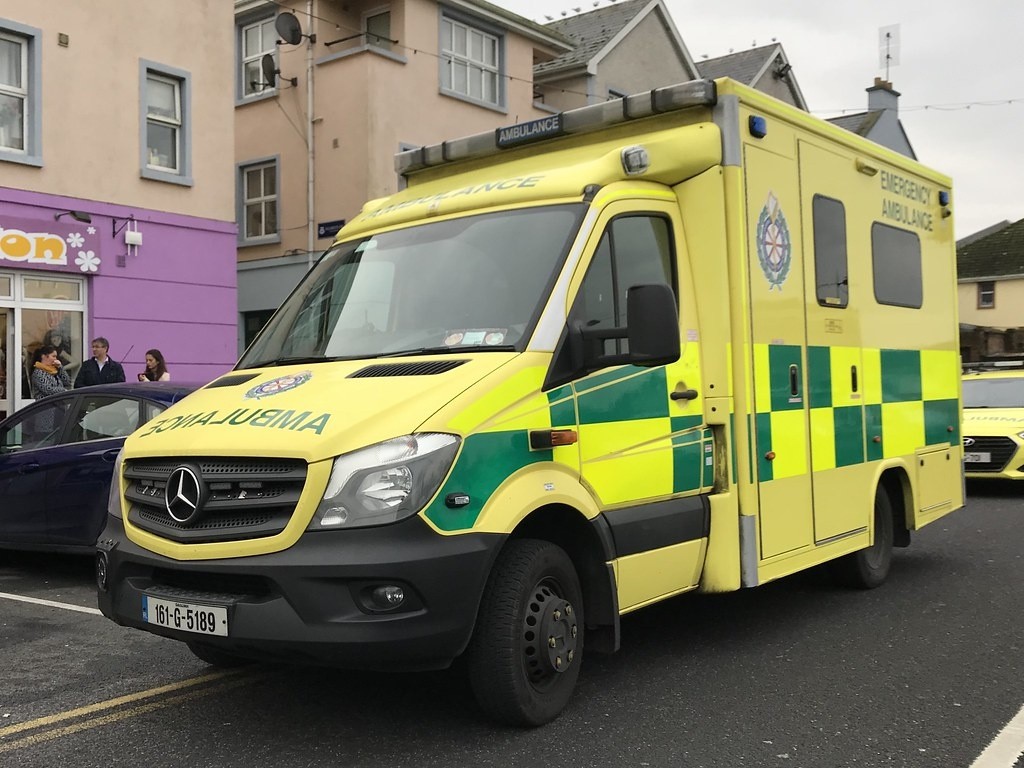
73, 336, 126, 390
30, 343, 72, 443
137, 349, 171, 382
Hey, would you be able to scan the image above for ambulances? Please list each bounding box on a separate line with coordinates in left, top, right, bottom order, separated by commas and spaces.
89, 75, 970, 729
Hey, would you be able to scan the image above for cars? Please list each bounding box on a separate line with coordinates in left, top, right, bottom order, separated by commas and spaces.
959, 372, 1024, 497
0, 380, 208, 572
